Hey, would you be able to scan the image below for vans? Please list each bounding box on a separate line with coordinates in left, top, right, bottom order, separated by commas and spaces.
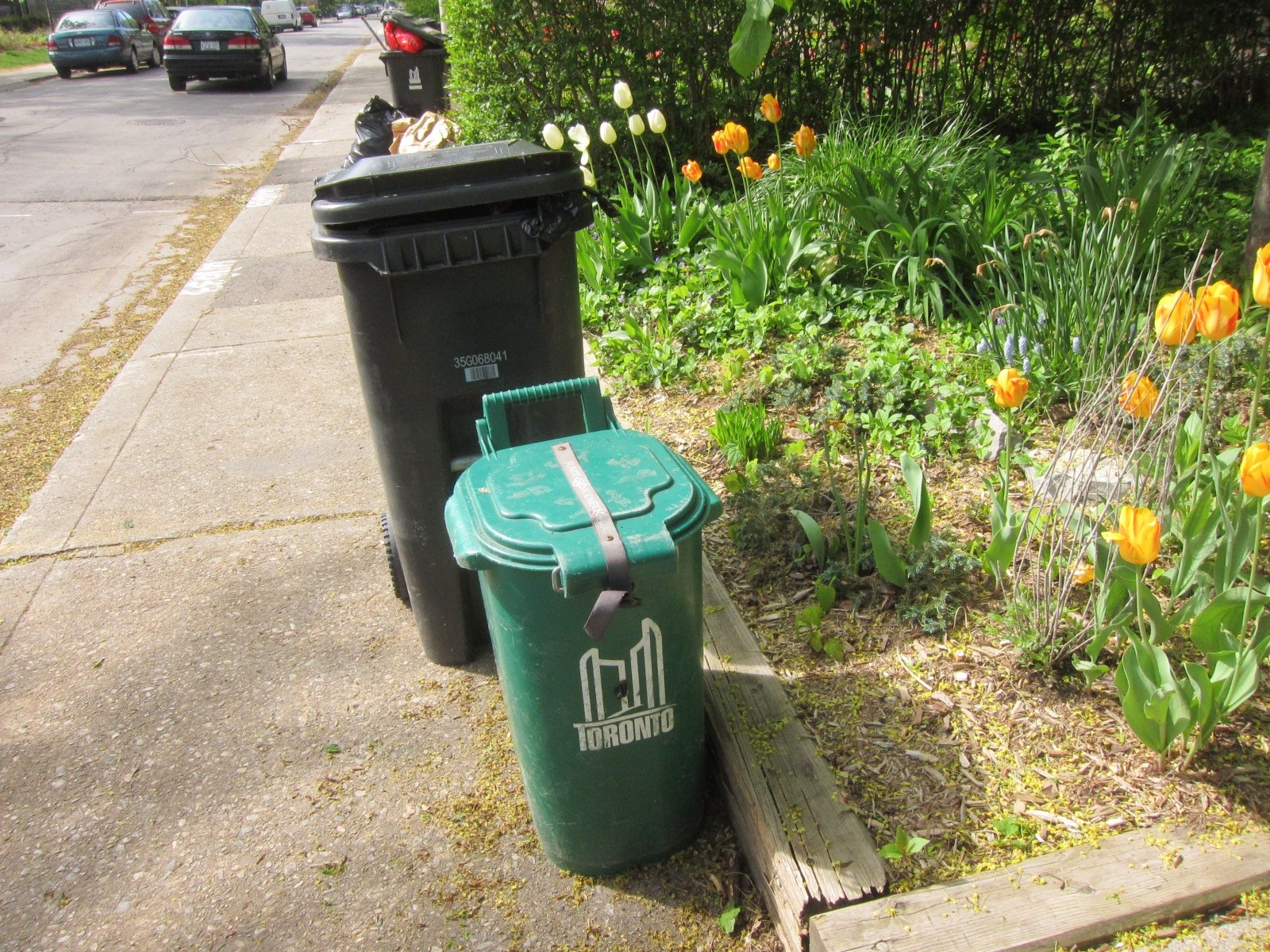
94, 0, 172, 53
261, 0, 305, 31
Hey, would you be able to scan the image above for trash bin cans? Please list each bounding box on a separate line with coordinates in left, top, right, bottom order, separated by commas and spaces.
308, 136, 593, 667
446, 377, 724, 878
379, 10, 452, 118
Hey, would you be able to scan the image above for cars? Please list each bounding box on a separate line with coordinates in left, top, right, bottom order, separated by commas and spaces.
162, 5, 288, 90
297, 6, 317, 26
47, 8, 164, 79
334, 4, 381, 19
